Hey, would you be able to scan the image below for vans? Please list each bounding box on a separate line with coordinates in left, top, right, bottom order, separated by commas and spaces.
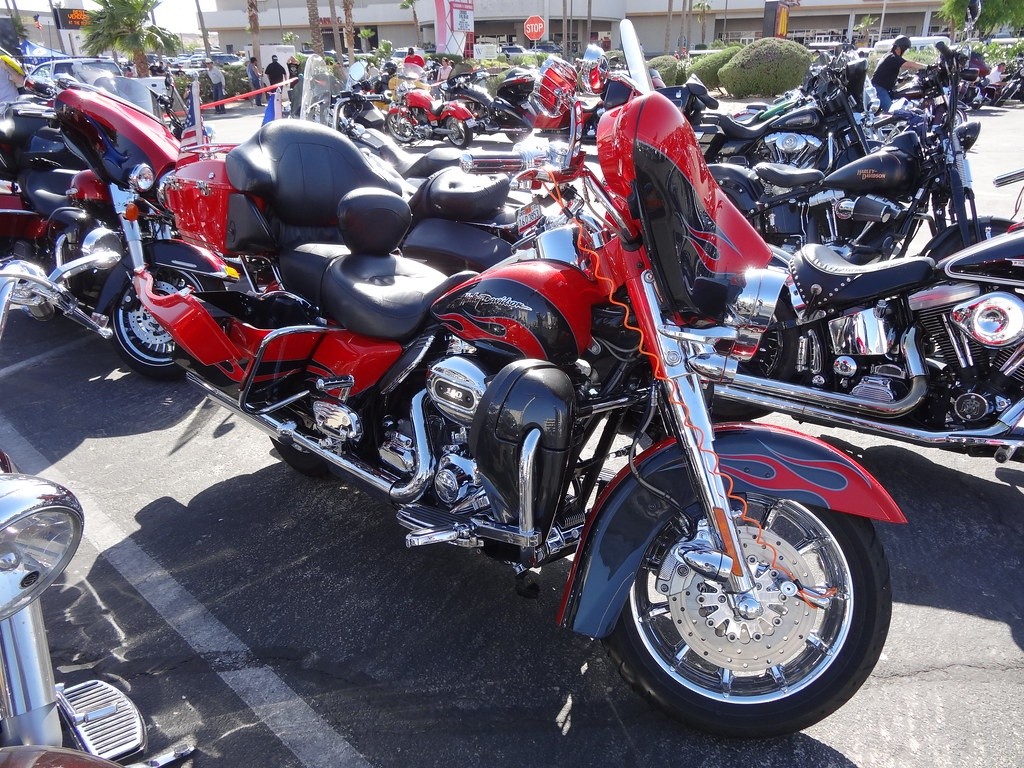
873, 36, 952, 54
389, 48, 426, 65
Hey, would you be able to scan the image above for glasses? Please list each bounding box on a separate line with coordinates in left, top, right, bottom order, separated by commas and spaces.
272, 58, 278, 61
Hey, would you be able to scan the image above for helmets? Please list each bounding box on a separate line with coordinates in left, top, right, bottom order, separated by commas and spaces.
383, 62, 397, 70
891, 35, 911, 48
407, 48, 414, 55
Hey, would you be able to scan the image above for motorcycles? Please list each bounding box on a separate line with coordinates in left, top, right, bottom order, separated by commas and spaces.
0, 1, 1024, 767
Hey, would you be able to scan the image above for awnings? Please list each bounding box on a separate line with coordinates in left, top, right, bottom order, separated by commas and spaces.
19, 39, 71, 65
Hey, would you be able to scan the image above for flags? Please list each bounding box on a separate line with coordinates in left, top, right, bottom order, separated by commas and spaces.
33, 13, 43, 31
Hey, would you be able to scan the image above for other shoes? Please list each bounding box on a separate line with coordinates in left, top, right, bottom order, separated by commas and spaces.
215, 109, 226, 114
257, 104, 265, 107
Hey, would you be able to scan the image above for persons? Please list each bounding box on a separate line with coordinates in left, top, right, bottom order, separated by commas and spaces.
265, 55, 286, 93
148, 60, 185, 78
871, 35, 928, 113
404, 47, 455, 82
247, 57, 265, 106
125, 67, 133, 78
204, 58, 226, 114
603, 37, 611, 51
985, 62, 1008, 106
118, 62, 122, 69
674, 51, 688, 61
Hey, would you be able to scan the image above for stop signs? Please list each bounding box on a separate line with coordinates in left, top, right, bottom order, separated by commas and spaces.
524, 15, 545, 40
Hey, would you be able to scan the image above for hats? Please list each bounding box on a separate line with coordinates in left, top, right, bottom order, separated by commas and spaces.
205, 58, 212, 62
